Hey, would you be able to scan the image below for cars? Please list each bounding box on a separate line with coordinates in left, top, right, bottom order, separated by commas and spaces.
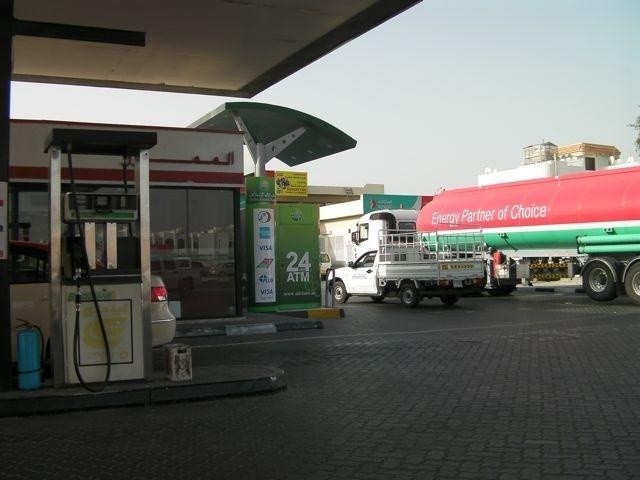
8, 240, 176, 377
139, 256, 235, 299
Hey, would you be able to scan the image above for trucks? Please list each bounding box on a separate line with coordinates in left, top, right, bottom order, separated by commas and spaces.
328, 229, 487, 306
351, 161, 640, 305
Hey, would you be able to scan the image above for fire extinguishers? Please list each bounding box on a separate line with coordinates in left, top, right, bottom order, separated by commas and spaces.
14, 318, 45, 391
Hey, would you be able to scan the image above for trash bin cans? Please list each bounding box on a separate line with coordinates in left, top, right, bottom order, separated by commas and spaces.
163, 343, 193, 382
